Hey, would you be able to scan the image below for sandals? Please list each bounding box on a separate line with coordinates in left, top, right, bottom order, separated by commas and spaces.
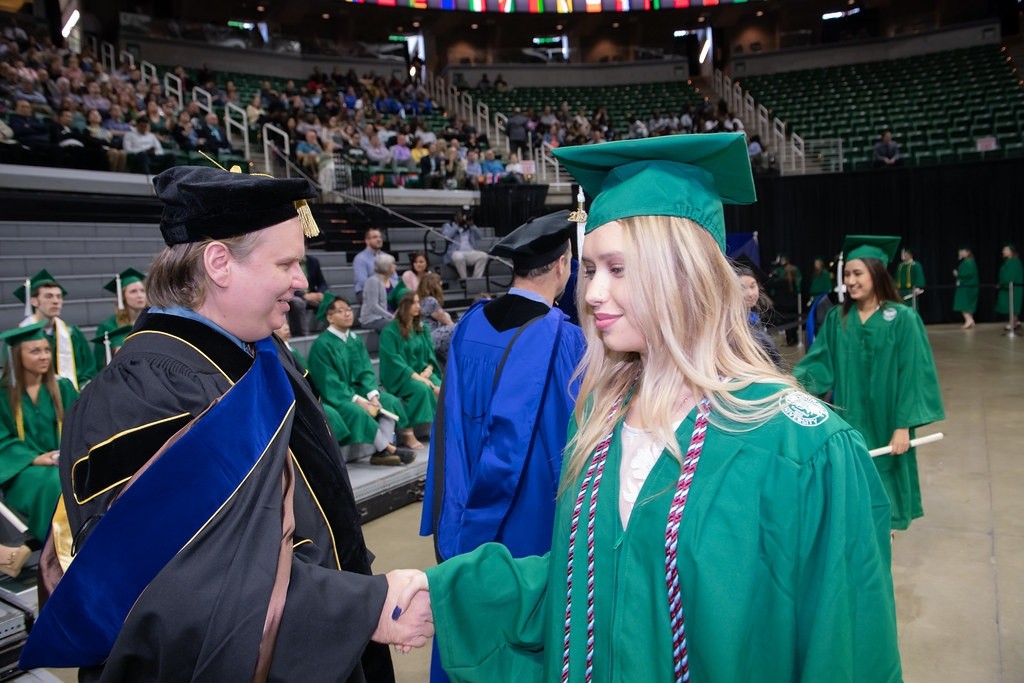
402, 431, 426, 451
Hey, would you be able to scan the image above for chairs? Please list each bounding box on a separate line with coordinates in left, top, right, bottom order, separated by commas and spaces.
0, 42, 1024, 187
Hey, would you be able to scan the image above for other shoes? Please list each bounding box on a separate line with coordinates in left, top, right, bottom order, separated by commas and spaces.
368, 451, 401, 466
395, 447, 417, 466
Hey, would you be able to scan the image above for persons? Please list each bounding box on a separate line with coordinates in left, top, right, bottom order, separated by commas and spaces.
953, 245, 979, 329
442, 208, 488, 278
0, 267, 148, 578
17, 166, 436, 683
420, 208, 590, 683
996, 244, 1024, 331
0, 18, 765, 190
772, 256, 834, 346
896, 247, 926, 310
872, 130, 900, 163
274, 228, 455, 469
792, 235, 947, 531
394, 133, 904, 683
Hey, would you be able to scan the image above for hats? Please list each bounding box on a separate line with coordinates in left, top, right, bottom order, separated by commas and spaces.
843, 233, 903, 274
1, 321, 51, 389
549, 121, 757, 256
386, 278, 410, 313
733, 261, 753, 279
103, 268, 148, 311
488, 210, 574, 270
151, 166, 318, 246
12, 269, 67, 318
315, 291, 337, 322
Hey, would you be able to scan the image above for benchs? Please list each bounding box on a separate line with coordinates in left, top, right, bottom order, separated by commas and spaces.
1, 219, 516, 652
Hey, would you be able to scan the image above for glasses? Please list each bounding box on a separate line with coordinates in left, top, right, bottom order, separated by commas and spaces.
328, 307, 351, 317
